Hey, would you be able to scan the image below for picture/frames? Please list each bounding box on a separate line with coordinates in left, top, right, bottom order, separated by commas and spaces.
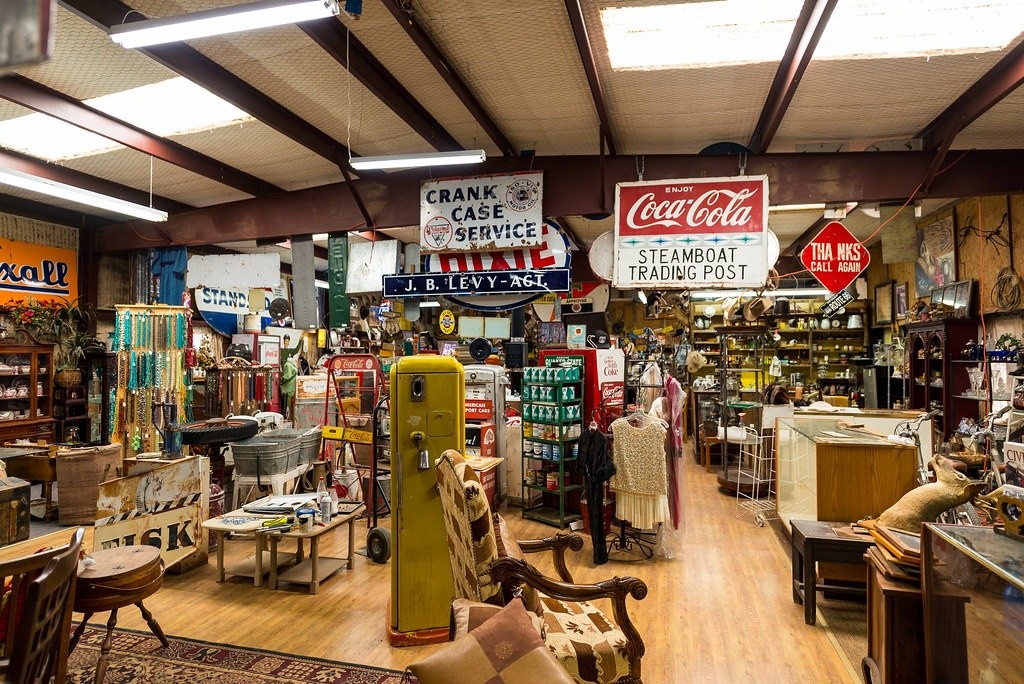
873, 279, 894, 324
893, 282, 909, 320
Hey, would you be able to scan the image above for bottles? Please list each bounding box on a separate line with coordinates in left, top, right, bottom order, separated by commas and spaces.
820, 318, 830, 329
296, 508, 315, 533
797, 318, 804, 329
322, 494, 332, 523
36, 382, 43, 396
330, 484, 338, 517
317, 477, 326, 510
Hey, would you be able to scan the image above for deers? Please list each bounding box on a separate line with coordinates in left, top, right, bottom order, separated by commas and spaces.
876, 453, 997, 534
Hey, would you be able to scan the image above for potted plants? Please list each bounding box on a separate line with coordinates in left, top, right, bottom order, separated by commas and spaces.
54, 294, 107, 387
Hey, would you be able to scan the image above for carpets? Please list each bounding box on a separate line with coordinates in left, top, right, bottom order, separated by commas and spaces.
68, 621, 417, 684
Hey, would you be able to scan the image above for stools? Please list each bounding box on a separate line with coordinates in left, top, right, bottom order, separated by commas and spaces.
66, 544, 169, 684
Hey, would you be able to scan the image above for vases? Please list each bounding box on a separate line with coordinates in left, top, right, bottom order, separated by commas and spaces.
28, 322, 55, 343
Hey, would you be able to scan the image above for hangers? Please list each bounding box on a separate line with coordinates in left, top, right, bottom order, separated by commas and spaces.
608, 398, 669, 432
589, 410, 602, 431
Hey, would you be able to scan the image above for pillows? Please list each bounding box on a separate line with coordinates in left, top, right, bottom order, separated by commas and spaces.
452, 599, 548, 644
405, 597, 576, 684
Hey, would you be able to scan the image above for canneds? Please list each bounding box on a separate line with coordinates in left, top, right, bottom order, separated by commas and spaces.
522, 366, 581, 491
299, 513, 313, 533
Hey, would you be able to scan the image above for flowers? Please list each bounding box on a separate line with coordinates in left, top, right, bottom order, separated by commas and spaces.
3, 299, 60, 325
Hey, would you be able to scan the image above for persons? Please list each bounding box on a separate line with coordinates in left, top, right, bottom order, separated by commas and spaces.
265, 328, 304, 372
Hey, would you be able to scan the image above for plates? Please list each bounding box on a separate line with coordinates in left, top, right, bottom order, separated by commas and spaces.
571, 303, 582, 313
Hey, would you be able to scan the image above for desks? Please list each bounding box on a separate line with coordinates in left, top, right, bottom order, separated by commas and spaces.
789, 520, 875, 625
230, 464, 317, 540
775, 417, 919, 598
201, 497, 367, 594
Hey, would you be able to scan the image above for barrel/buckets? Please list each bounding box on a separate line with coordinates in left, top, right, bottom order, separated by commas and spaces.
223, 437, 301, 475
259, 428, 322, 464
333, 469, 358, 501
208, 482, 225, 554
363, 473, 391, 515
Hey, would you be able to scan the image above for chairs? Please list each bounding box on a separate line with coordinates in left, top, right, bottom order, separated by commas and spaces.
437, 450, 647, 684
0, 528, 86, 684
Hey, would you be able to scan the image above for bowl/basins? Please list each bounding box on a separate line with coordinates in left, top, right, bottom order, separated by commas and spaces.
19, 366, 30, 373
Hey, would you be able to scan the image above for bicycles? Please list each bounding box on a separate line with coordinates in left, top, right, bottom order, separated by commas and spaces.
893, 405, 984, 529
968, 404, 1024, 536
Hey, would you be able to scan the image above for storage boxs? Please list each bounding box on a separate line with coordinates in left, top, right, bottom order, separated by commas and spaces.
56, 442, 122, 525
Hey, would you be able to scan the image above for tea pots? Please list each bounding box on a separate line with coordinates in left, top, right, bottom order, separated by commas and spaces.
694, 318, 703, 328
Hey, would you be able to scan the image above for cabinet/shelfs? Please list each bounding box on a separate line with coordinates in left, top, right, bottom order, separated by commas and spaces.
0, 343, 53, 427
950, 359, 1018, 402
520, 378, 585, 529
901, 316, 978, 441
53, 385, 89, 443
687, 298, 870, 463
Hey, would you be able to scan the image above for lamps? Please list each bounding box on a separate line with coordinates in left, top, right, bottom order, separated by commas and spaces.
0, 0, 489, 222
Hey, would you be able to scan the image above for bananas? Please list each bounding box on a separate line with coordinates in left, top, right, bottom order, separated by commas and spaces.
262, 517, 288, 527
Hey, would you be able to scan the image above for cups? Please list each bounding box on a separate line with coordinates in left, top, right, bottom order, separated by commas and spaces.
37, 408, 40, 415
14, 411, 20, 415
0, 410, 14, 421
704, 320, 709, 328
840, 345, 853, 364
812, 343, 829, 364
40, 368, 46, 373
17, 388, 26, 392
24, 409, 30, 417
795, 386, 804, 400
5, 389, 13, 397
